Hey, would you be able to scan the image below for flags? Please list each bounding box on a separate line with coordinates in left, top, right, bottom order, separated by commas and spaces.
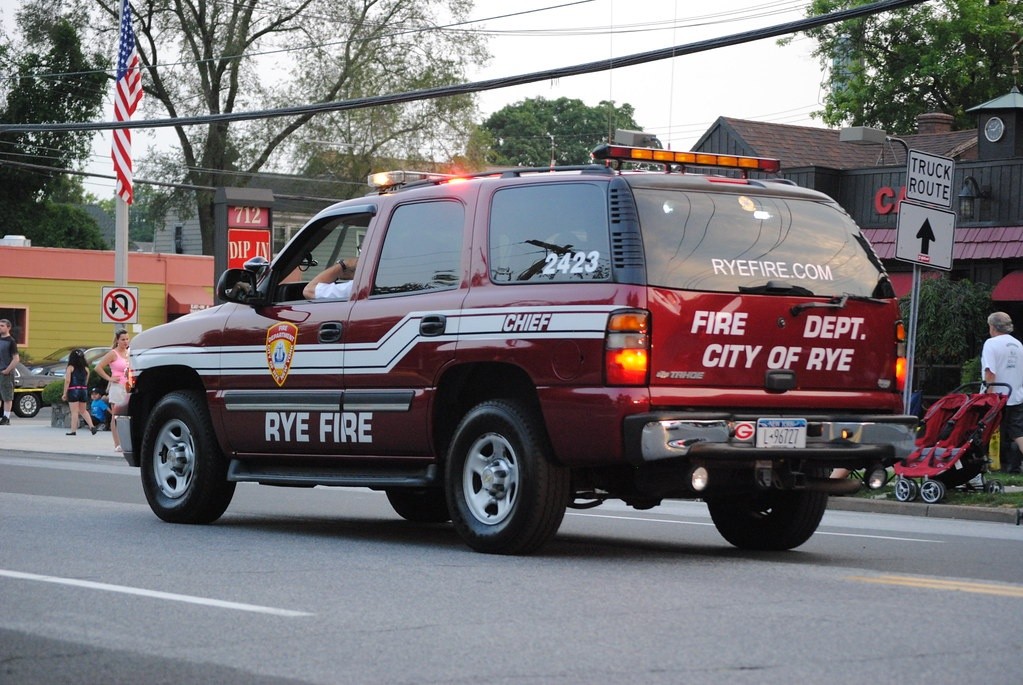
109, 0, 144, 205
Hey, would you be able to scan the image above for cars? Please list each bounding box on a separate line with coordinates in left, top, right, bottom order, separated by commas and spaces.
13, 346, 112, 418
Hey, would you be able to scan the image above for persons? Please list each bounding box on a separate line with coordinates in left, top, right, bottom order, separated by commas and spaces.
0, 319, 20, 425
94, 328, 131, 452
78, 369, 113, 431
979, 311, 1023, 475
302, 245, 364, 301
62, 349, 97, 435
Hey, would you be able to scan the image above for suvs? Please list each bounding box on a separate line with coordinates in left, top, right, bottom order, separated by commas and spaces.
839, 127, 921, 417
109, 129, 917, 553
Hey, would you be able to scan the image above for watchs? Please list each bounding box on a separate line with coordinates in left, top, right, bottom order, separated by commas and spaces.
336, 259, 347, 272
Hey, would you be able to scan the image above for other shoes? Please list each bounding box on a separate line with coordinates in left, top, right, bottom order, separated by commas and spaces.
66, 432, 76, 435
114, 445, 121, 452
0, 415, 9, 425
90, 426, 97, 434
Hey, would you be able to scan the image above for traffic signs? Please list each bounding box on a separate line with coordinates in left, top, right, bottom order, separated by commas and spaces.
895, 148, 955, 272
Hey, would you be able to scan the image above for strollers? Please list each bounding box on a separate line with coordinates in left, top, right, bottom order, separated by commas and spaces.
893, 380, 1012, 503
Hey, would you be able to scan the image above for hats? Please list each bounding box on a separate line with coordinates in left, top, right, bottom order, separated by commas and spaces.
89, 387, 99, 393
